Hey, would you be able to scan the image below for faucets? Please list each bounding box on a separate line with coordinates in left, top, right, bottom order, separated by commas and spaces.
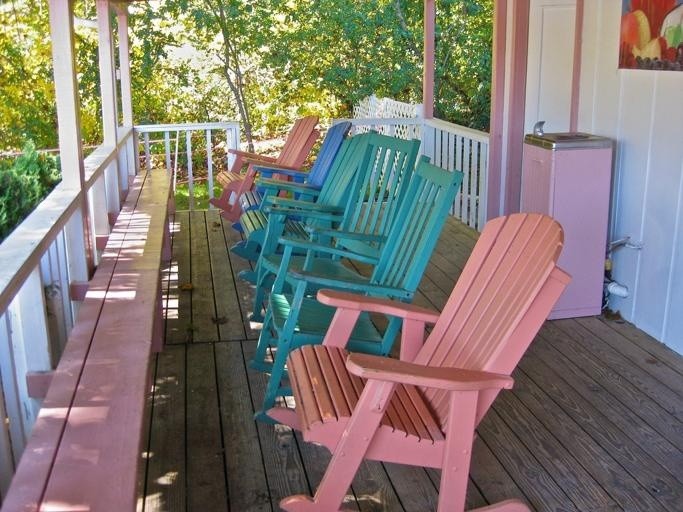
534, 121, 545, 137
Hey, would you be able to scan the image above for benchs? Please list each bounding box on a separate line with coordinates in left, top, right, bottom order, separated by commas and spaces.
0, 169, 174, 512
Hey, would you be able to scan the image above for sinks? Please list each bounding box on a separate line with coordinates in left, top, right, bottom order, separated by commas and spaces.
551, 132, 611, 148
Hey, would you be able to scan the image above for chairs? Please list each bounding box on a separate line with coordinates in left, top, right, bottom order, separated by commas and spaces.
267, 212, 573, 512
207, 113, 466, 432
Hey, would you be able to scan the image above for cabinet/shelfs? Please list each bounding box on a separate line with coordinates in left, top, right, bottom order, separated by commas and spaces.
515, 121, 615, 319
